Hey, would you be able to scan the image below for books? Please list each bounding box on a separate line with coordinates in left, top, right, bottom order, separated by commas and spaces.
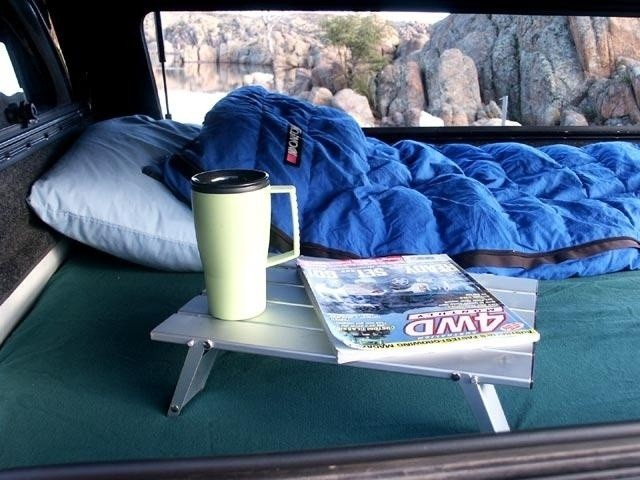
297, 253, 540, 365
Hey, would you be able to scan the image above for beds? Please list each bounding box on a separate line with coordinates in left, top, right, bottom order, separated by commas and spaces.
0, 248, 638, 480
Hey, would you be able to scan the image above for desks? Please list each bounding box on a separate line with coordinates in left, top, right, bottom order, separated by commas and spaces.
150, 252, 539, 433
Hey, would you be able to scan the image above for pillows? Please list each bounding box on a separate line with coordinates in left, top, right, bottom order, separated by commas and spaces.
25, 114, 203, 272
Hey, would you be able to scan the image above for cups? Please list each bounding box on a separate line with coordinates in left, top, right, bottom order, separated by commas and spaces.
191, 166, 301, 323
